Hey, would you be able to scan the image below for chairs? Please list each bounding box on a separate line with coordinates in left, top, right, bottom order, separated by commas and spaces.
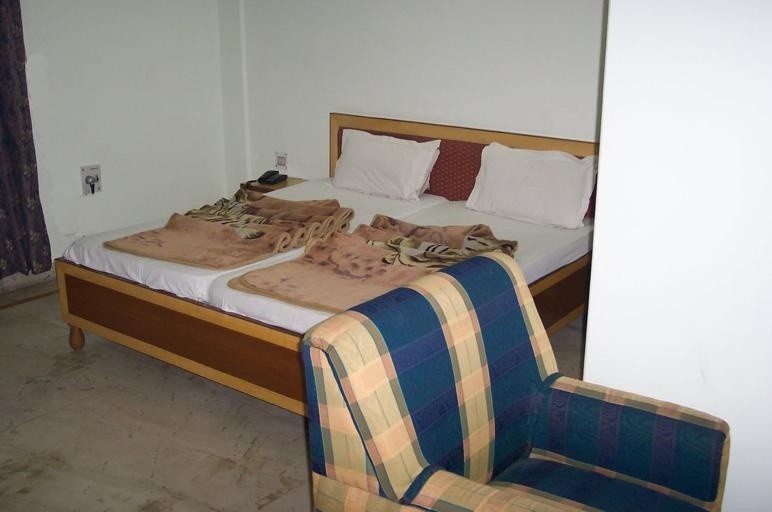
300, 250, 730, 512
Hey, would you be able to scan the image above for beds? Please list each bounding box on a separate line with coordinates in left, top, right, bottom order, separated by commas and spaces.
53, 113, 598, 420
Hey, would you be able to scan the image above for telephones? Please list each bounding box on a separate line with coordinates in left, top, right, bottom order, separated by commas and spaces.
258, 170, 287, 185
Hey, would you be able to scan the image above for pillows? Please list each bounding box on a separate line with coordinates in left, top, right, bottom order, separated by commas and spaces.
464, 140, 599, 229
329, 128, 441, 204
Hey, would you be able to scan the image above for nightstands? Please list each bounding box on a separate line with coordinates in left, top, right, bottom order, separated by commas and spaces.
240, 177, 308, 192
